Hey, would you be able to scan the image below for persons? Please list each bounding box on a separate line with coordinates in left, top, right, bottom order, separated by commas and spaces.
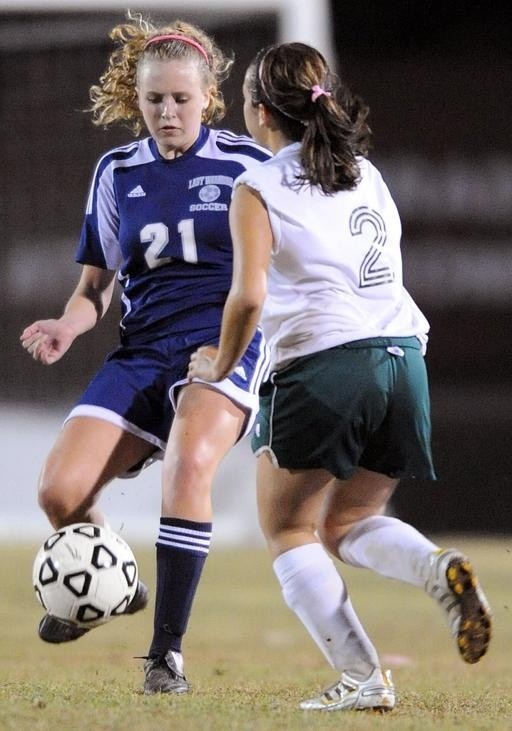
18, 8, 276, 703
181, 33, 496, 712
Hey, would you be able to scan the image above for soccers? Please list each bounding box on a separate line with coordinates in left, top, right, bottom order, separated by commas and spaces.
33, 522, 138, 628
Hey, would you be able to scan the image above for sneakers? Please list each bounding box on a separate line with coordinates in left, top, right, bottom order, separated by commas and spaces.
421, 549, 491, 664
37, 580, 146, 643
298, 669, 397, 712
143, 650, 188, 697
